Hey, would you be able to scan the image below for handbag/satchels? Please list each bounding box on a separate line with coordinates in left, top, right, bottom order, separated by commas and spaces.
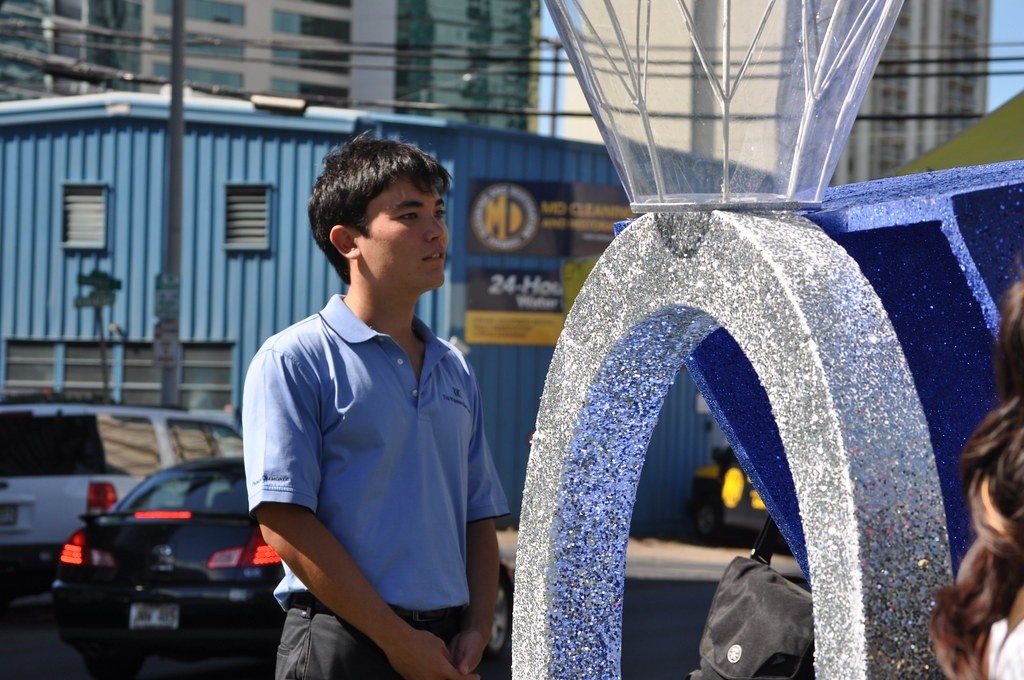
699, 558, 813, 680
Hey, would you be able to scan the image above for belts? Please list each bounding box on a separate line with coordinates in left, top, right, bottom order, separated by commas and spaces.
289, 592, 469, 622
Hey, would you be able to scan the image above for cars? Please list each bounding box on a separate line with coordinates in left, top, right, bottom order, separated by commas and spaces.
49, 456, 520, 674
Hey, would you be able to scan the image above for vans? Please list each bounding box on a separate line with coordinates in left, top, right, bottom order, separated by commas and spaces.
690, 445, 793, 557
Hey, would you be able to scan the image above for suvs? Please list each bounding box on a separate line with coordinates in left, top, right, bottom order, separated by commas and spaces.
0, 390, 246, 609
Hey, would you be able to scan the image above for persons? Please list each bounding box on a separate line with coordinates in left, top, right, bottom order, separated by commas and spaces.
242, 140, 500, 680
931, 275, 1024, 680
